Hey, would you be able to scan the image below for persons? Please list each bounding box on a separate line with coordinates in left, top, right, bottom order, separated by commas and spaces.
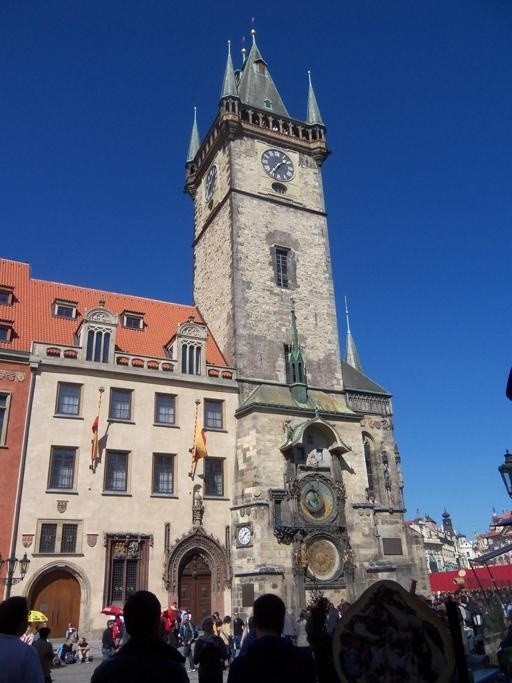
2, 596, 91, 683
92, 585, 512, 683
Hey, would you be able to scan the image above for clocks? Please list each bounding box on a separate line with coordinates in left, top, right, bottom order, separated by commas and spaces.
203, 164, 220, 203
235, 521, 254, 549
262, 148, 295, 183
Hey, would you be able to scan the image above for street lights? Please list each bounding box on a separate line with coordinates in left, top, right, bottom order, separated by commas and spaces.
1, 550, 30, 600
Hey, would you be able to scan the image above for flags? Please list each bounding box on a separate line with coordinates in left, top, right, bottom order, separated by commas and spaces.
193, 411, 207, 463
92, 414, 99, 459
